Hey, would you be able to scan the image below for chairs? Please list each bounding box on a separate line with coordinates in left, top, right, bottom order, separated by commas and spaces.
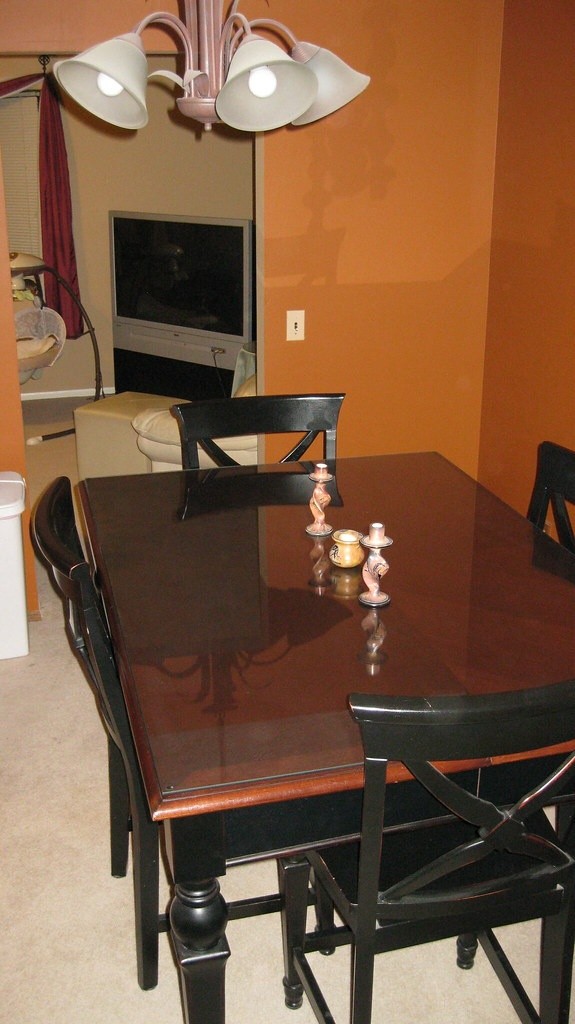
25, 387, 574, 1023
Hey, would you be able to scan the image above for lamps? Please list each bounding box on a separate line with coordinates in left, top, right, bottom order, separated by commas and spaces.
96, 67, 280, 100
